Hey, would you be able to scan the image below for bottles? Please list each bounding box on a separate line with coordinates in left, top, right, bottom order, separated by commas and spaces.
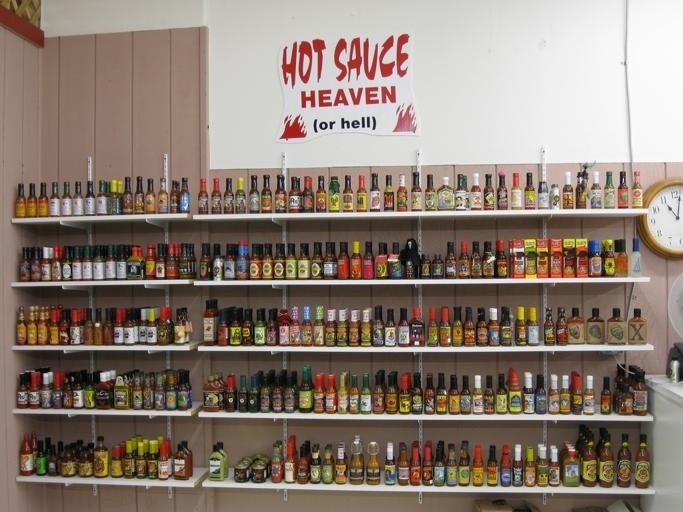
628, 308, 648, 344
482, 241, 495, 279
590, 171, 602, 208
510, 172, 522, 209
515, 306, 526, 346
296, 178, 304, 207
307, 367, 314, 411
244, 241, 250, 279
617, 433, 632, 488
313, 304, 325, 346
298, 367, 312, 413
48, 182, 60, 217
547, 444, 557, 465
411, 172, 423, 211
96, 179, 109, 215
604, 171, 616, 208
208, 243, 213, 281
266, 309, 278, 345
524, 172, 536, 209
559, 424, 614, 488
18, 242, 197, 281
425, 174, 438, 211
93, 435, 109, 478
567, 307, 586, 344
607, 307, 628, 344
181, 440, 193, 476
618, 170, 629, 209
217, 441, 229, 478
310, 177, 314, 212
431, 253, 444, 279
384, 439, 446, 486
576, 172, 586, 208
371, 306, 385, 346
362, 240, 374, 280
447, 374, 460, 415
278, 309, 289, 346
110, 179, 120, 214
405, 241, 416, 279
338, 242, 350, 280
495, 239, 508, 278
297, 243, 311, 279
615, 239, 629, 278
118, 180, 125, 214
437, 177, 455, 211
375, 242, 388, 279
211, 178, 222, 214
601, 376, 611, 414
527, 307, 540, 346
200, 243, 208, 281
507, 368, 522, 414
242, 307, 253, 346
110, 434, 172, 478
446, 443, 458, 486
583, 375, 595, 415
44, 437, 51, 460
56, 440, 65, 475
356, 175, 367, 212
61, 181, 72, 216
253, 308, 266, 345
461, 440, 470, 463
635, 434, 650, 488
145, 179, 156, 214
476, 307, 489, 346
134, 176, 145, 214
470, 173, 482, 210
31, 432, 39, 473
203, 370, 226, 412
397, 307, 410, 347
548, 374, 561, 414
249, 175, 260, 213
77, 440, 83, 474
426, 306, 439, 347
275, 175, 287, 213
238, 375, 248, 413
38, 182, 49, 217
359, 373, 372, 415
536, 444, 545, 483
311, 242, 324, 280
550, 184, 561, 210
372, 369, 435, 414
79, 446, 94, 477
270, 434, 365, 485
571, 371, 583, 414
471, 241, 482, 278
436, 373, 447, 414
88, 441, 95, 456
455, 174, 467, 211
588, 239, 616, 277
250, 240, 287, 279
16, 368, 192, 411
445, 242, 457, 278
484, 375, 496, 415
235, 176, 246, 214
562, 172, 574, 209
370, 173, 380, 212
173, 444, 190, 480
324, 242, 335, 280
463, 307, 477, 346
536, 445, 549, 487
303, 175, 313, 213
473, 444, 484, 486
472, 375, 484, 415
15, 305, 193, 346
289, 177, 302, 212
669, 356, 681, 384
301, 304, 314, 345
349, 240, 362, 280
522, 371, 535, 414
484, 173, 495, 210
452, 306, 463, 347
538, 182, 550, 209
314, 369, 351, 414
409, 307, 426, 346
61, 444, 76, 477
36, 440, 47, 476
331, 242, 338, 280
500, 445, 512, 486
397, 170, 408, 212
383, 175, 394, 212
366, 441, 380, 485
224, 243, 238, 280
328, 175, 340, 212
342, 175, 353, 212
84, 181, 96, 216
457, 240, 471, 279
20, 432, 35, 476
512, 444, 524, 487
289, 303, 301, 346
629, 238, 643, 277
524, 447, 537, 486
548, 447, 560, 486
500, 307, 513, 347
198, 179, 208, 214
613, 363, 648, 416
586, 308, 605, 344
488, 307, 500, 346
70, 442, 77, 476
463, 176, 470, 211
224, 177, 234, 214
535, 374, 546, 414
46, 445, 59, 477
439, 307, 453, 347
544, 307, 556, 346
26, 183, 38, 217
420, 254, 431, 279
556, 307, 568, 344
236, 240, 245, 280
316, 176, 327, 213
203, 298, 243, 346
385, 308, 397, 347
122, 176, 133, 215
158, 178, 168, 213
107, 181, 112, 215
15, 183, 26, 217
179, 178, 191, 213
213, 243, 223, 280
458, 448, 470, 486
286, 243, 298, 280
170, 180, 179, 213
461, 376, 471, 415
495, 373, 508, 414
349, 374, 360, 414
388, 242, 402, 279
560, 375, 571, 414
248, 369, 299, 413
325, 307, 371, 346
226, 373, 237, 412
487, 446, 498, 486
632, 171, 644, 208
261, 175, 272, 213
72, 181, 84, 216
208, 444, 225, 481
497, 172, 508, 210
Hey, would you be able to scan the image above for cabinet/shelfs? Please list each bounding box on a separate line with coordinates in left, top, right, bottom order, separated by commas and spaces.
192, 209, 658, 496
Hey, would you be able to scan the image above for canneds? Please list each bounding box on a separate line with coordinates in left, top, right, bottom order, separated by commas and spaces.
233, 453, 271, 482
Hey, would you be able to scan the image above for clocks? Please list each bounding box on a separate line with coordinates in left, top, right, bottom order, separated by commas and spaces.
636, 179, 683, 259
